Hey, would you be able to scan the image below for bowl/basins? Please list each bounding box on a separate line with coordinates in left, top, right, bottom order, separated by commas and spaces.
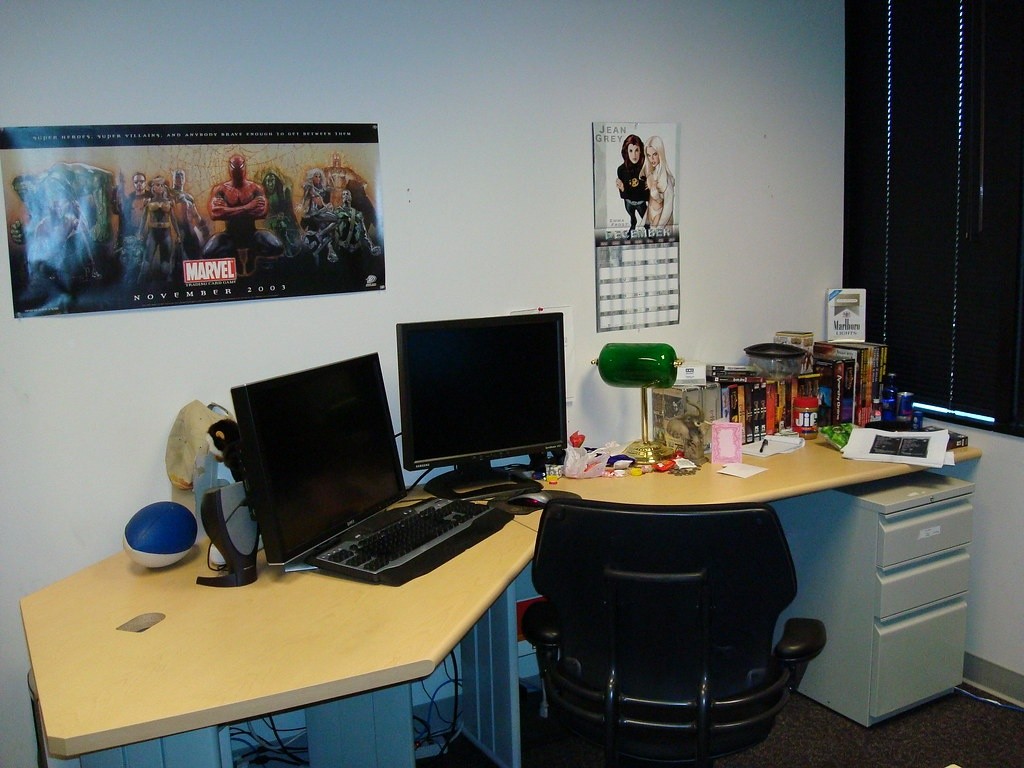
745, 343, 806, 381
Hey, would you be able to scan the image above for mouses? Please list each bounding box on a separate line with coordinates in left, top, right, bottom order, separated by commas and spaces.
508, 486, 552, 507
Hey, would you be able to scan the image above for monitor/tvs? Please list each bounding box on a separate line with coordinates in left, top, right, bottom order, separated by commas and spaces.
396, 312, 568, 500
229, 352, 408, 571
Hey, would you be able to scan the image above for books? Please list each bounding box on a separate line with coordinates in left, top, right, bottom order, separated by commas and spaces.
651, 289, 968, 458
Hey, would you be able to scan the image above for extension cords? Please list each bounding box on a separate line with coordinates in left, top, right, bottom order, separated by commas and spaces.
415, 736, 448, 760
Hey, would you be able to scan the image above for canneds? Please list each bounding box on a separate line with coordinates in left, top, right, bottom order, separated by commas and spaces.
895, 392, 923, 431
793, 397, 819, 440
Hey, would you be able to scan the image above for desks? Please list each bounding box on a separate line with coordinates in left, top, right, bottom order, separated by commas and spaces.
19, 484, 539, 768
462, 428, 983, 768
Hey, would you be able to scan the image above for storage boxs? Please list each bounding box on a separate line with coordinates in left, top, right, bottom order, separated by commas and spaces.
825, 288, 866, 344
774, 331, 813, 373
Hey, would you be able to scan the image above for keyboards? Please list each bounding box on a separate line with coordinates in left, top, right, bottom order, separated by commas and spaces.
303, 498, 514, 587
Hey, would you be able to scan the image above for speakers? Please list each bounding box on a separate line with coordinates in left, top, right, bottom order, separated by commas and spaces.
196, 481, 261, 587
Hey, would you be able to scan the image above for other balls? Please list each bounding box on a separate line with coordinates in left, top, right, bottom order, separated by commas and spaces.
124, 501, 198, 568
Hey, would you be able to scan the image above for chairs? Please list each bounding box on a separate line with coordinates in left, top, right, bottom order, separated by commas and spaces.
521, 497, 828, 768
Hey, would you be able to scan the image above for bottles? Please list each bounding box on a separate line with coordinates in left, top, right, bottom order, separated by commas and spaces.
880, 374, 897, 421
